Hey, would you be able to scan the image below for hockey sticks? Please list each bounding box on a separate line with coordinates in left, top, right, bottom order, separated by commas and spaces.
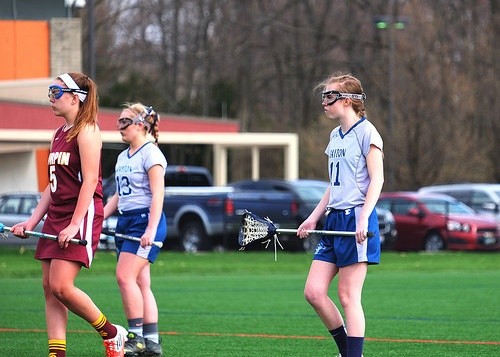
0, 223, 89, 246
238, 209, 373, 248
101, 229, 163, 246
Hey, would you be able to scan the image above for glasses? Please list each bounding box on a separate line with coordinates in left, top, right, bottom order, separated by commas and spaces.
47, 84, 89, 100
320, 89, 367, 105
116, 108, 150, 129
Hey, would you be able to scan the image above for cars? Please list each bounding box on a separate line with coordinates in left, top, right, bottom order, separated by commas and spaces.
420, 183, 500, 218
226, 179, 398, 251
375, 191, 500, 253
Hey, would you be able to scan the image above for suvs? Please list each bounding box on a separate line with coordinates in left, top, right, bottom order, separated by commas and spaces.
0, 192, 119, 250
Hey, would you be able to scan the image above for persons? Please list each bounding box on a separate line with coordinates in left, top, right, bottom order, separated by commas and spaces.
104, 102, 168, 357
297, 74, 385, 357
10, 72, 128, 357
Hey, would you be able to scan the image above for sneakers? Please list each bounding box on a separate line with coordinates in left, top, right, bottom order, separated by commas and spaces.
102, 323, 129, 357
123, 331, 146, 357
138, 337, 163, 357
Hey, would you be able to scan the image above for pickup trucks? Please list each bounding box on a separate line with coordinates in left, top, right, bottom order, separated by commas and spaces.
102, 165, 300, 251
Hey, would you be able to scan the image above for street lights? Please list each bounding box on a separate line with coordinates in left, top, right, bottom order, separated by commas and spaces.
374, 16, 408, 187
